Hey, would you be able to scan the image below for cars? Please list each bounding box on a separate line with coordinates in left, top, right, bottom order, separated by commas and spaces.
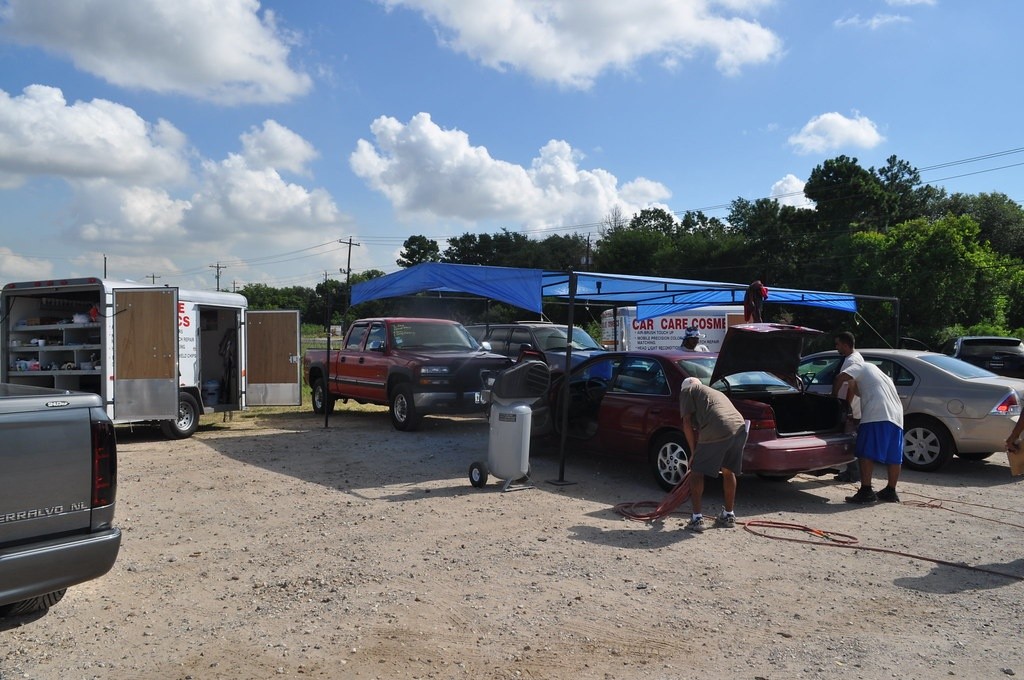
797, 348, 1024, 473
489, 322, 856, 491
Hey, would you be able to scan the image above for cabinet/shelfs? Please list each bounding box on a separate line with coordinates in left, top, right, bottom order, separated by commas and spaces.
8, 322, 101, 376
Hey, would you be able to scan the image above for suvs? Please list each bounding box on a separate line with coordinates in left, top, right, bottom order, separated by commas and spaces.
897, 335, 1024, 380
463, 322, 607, 377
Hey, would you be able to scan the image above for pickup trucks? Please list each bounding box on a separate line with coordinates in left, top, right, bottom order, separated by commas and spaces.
1, 382, 122, 618
301, 317, 513, 432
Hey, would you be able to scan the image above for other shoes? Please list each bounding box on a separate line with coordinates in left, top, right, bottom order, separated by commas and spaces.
845, 488, 875, 503
875, 486, 896, 501
834, 470, 861, 482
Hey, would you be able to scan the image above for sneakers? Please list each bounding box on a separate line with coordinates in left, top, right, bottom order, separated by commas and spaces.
715, 505, 736, 528
688, 516, 707, 531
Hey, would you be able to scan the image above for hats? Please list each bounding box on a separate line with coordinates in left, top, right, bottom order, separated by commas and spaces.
682, 329, 705, 338
681, 377, 702, 391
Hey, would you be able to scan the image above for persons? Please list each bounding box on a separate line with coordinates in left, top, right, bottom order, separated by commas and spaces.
676, 327, 709, 352
680, 376, 747, 530
834, 331, 864, 483
832, 361, 904, 505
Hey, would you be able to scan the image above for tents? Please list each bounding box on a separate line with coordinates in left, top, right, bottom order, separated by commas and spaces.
350, 263, 856, 487
